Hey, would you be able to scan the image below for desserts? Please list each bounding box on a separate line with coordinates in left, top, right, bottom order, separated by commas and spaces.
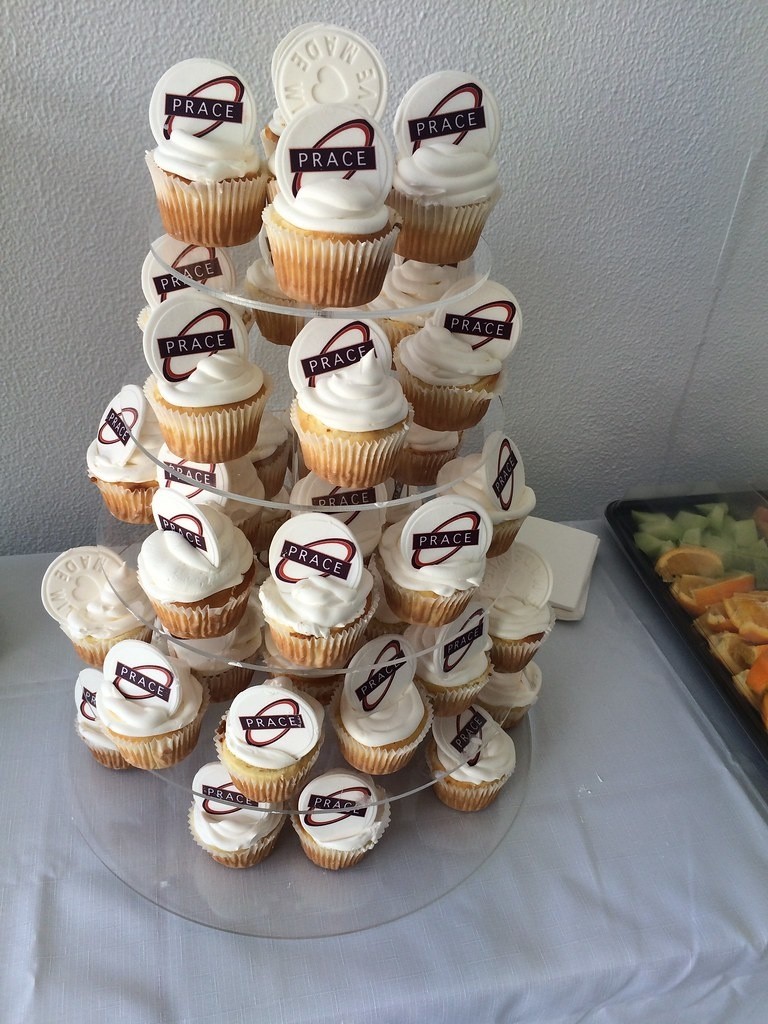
58, 112, 552, 868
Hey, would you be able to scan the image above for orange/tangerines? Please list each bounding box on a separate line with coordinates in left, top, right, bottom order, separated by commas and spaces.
658, 550, 768, 731
